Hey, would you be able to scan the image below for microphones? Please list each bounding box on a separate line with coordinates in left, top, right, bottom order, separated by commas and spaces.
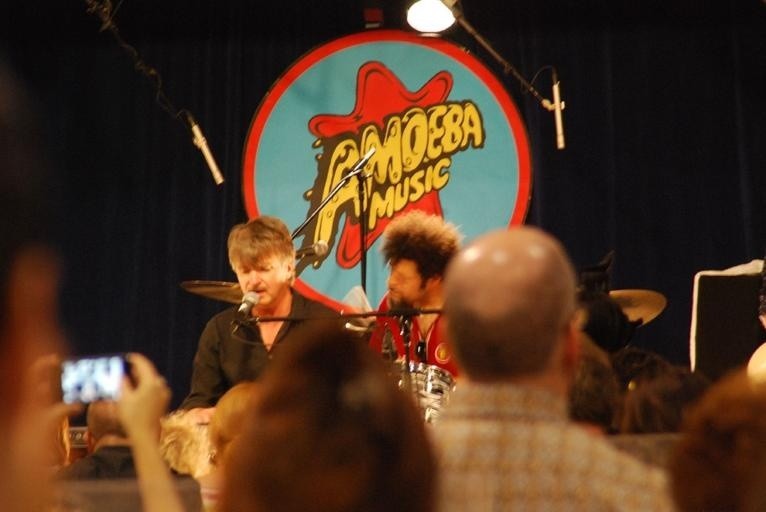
236, 290, 261, 320
294, 240, 328, 260
552, 82, 570, 152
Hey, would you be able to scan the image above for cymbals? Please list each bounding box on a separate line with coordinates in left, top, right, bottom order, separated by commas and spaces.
610, 288, 667, 331
181, 280, 245, 306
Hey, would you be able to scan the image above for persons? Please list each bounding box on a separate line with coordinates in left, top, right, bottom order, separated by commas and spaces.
567, 256, 766, 512
0, 230, 189, 512
178, 215, 346, 425
193, 380, 257, 511
215, 313, 439, 512
426, 223, 680, 512
364, 209, 463, 423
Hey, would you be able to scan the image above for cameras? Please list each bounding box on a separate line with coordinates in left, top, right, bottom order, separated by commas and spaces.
56, 354, 127, 408
